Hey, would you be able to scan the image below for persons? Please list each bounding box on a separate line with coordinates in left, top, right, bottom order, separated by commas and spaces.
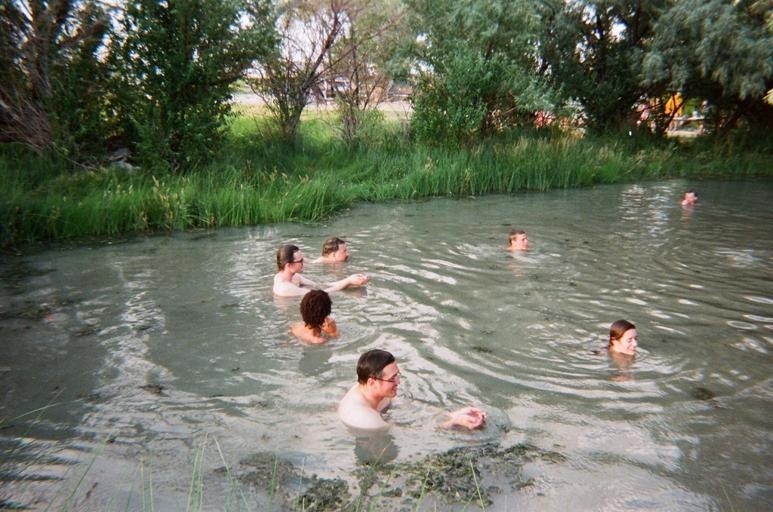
508, 229, 530, 251
609, 320, 639, 357
337, 349, 487, 430
316, 238, 349, 266
681, 190, 698, 207
273, 246, 369, 298
290, 291, 340, 344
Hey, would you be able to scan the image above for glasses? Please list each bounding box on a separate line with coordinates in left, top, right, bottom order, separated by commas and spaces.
376, 371, 402, 383
289, 257, 303, 264
